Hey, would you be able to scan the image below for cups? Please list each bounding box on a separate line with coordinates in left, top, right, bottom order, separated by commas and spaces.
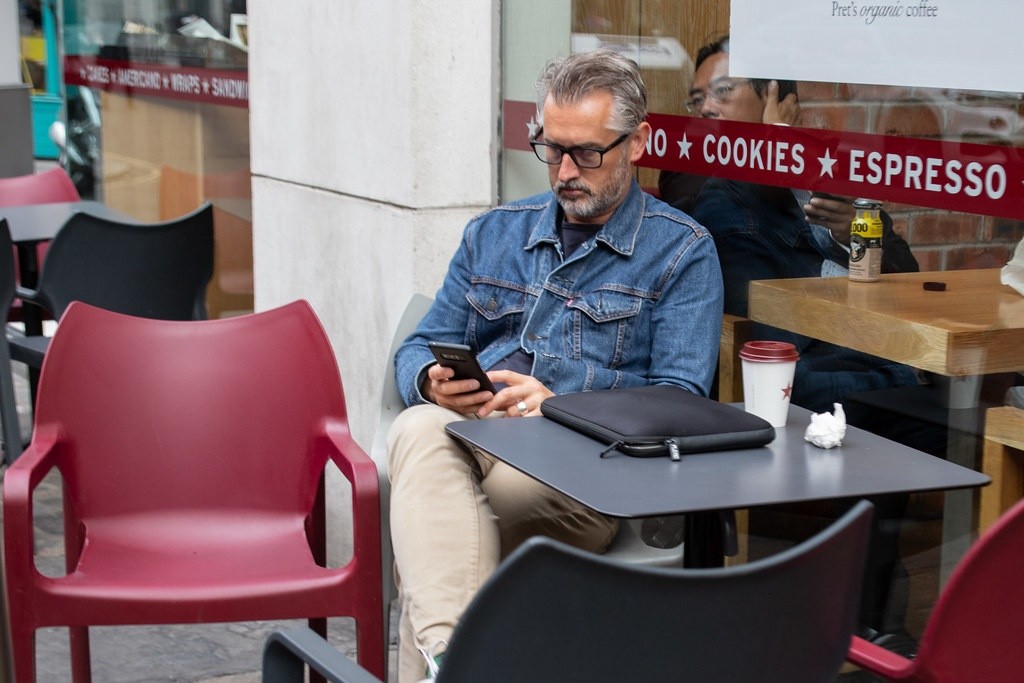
738, 340, 799, 428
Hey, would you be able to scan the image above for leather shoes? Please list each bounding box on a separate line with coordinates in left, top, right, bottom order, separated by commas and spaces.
865, 626, 920, 660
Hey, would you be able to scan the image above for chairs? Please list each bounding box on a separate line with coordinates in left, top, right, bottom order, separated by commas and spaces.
0, 167, 1024, 683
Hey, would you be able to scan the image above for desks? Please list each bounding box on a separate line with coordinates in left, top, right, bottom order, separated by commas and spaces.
0, 198, 146, 438
443, 403, 993, 570
750, 268, 1024, 599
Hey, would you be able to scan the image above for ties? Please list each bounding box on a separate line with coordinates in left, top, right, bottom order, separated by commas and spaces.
789, 186, 834, 254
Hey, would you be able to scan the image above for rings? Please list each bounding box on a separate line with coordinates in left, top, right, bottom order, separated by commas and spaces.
516, 401, 529, 416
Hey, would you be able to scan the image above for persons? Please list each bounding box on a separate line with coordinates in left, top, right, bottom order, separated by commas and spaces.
656, 36, 924, 659
383, 52, 722, 683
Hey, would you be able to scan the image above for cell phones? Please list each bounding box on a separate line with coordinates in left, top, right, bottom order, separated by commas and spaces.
427, 342, 496, 395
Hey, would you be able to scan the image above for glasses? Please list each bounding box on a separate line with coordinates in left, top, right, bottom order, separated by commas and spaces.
685, 79, 757, 114
529, 127, 629, 169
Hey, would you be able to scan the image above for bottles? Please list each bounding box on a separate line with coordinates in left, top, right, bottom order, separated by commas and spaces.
848, 196, 883, 282
